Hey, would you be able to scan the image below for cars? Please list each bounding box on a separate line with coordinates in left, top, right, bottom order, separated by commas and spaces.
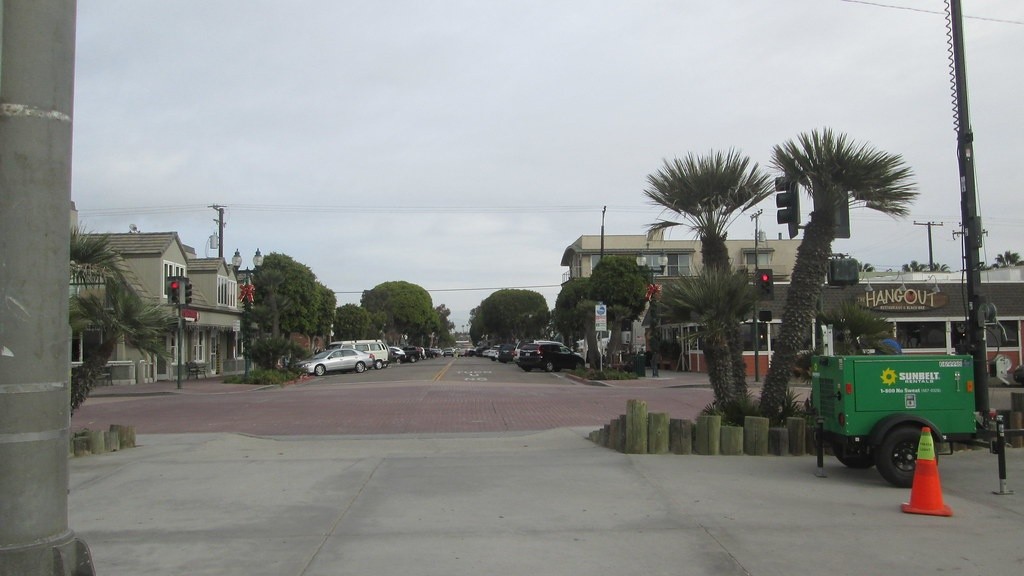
401, 339, 553, 363
390, 346, 408, 365
296, 347, 375, 376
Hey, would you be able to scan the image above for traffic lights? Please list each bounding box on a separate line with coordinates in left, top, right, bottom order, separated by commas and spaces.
167, 276, 181, 305
184, 277, 193, 305
755, 269, 774, 301
775, 170, 800, 239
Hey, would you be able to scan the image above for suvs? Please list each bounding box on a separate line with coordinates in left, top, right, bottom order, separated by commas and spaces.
519, 341, 585, 372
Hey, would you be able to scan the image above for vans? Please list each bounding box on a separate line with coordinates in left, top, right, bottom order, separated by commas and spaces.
326, 339, 390, 370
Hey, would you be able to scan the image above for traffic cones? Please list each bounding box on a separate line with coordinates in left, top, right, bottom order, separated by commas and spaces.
901, 426, 953, 517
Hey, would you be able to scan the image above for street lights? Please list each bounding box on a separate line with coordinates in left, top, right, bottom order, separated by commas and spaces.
231, 247, 263, 372
636, 247, 669, 377
